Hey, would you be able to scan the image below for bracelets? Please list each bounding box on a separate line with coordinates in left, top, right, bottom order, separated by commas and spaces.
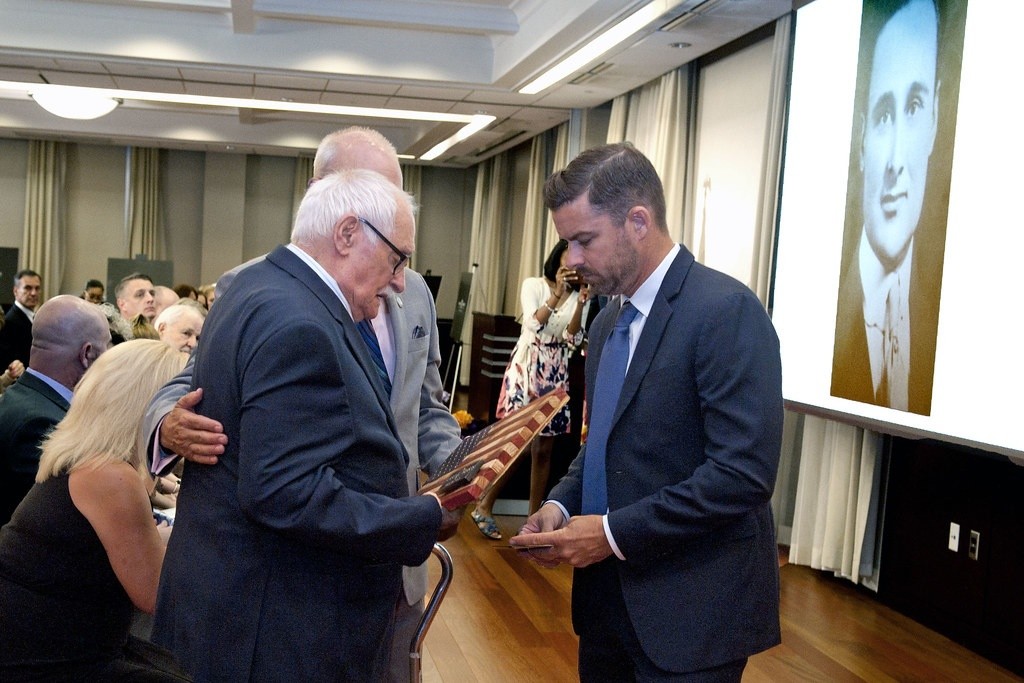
544, 301, 554, 311
554, 292, 561, 299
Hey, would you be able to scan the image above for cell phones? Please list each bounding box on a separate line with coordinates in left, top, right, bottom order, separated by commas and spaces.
567, 274, 586, 284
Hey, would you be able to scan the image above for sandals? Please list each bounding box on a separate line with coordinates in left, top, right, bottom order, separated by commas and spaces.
470, 507, 502, 540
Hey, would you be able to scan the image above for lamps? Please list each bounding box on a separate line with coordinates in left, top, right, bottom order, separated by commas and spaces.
28, 83, 124, 122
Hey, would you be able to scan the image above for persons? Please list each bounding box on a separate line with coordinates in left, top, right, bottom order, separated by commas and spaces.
472, 239, 591, 540
833, 0, 949, 417
203, 287, 215, 310
0, 295, 111, 528
0, 360, 26, 396
155, 298, 210, 355
0, 269, 43, 376
509, 143, 782, 683
0, 336, 193, 683
139, 126, 465, 683
84, 280, 104, 304
140, 170, 465, 683
174, 285, 198, 301
115, 273, 157, 325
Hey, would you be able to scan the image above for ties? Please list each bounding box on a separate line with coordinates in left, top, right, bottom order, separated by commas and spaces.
356, 317, 393, 402
580, 302, 639, 516
885, 270, 907, 413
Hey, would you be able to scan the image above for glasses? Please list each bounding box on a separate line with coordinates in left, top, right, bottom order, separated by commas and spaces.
357, 216, 411, 276
